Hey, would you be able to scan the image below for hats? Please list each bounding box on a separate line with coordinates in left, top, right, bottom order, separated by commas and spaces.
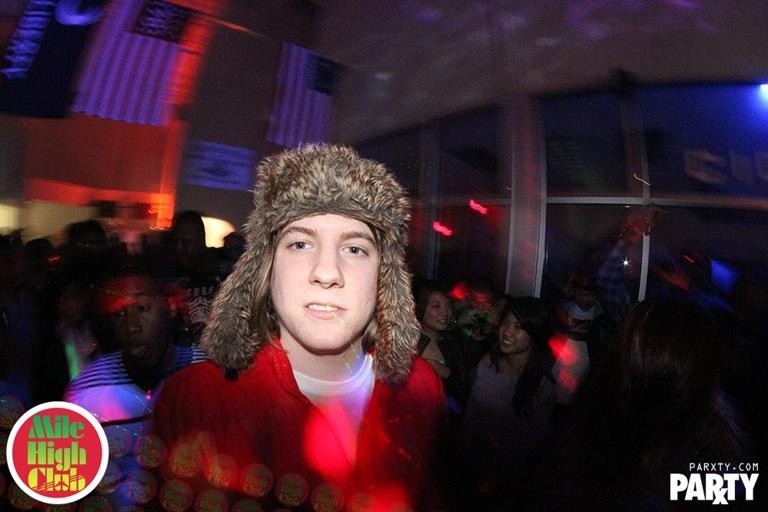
199, 143, 422, 385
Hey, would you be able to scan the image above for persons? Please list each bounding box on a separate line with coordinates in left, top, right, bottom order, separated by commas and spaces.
0, 142, 768, 512
